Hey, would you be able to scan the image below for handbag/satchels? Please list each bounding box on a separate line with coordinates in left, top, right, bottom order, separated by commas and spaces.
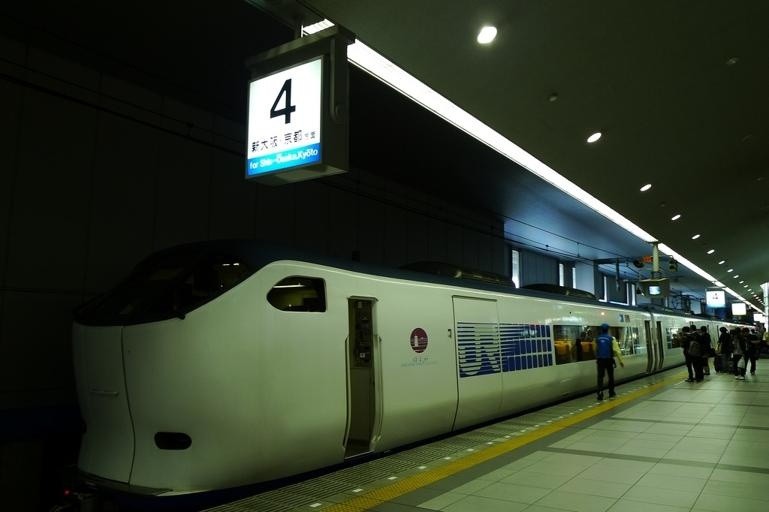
709, 347, 716, 357
737, 357, 745, 368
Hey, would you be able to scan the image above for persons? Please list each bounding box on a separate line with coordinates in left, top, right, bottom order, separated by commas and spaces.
671, 323, 769, 382
575, 330, 593, 361
592, 322, 624, 400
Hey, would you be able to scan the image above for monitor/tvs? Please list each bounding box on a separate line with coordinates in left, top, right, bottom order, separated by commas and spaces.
649, 285, 661, 295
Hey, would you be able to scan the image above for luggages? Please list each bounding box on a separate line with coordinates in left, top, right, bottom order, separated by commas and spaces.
714, 345, 727, 373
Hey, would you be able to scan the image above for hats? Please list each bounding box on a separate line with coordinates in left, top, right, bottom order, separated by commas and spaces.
600, 323, 609, 329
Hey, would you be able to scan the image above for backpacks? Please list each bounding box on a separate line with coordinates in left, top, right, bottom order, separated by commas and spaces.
684, 333, 699, 356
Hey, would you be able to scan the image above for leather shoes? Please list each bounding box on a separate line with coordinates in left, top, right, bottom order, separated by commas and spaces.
609, 391, 616, 398
597, 391, 603, 400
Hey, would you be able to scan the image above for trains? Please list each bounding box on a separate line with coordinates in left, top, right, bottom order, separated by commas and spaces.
62, 241, 764, 506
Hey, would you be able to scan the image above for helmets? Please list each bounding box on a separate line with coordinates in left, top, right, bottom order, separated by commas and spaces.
720, 327, 728, 331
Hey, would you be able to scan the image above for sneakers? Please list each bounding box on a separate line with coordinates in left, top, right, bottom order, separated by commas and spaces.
734, 375, 745, 380
684, 371, 710, 382
